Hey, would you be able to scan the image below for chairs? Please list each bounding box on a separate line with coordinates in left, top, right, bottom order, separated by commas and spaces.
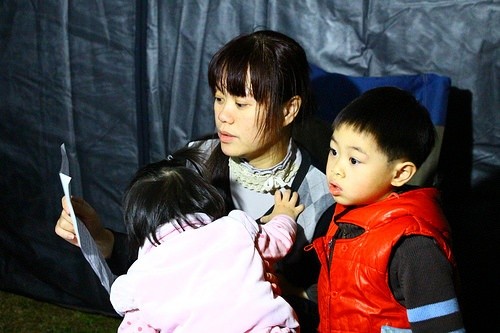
304, 62, 451, 186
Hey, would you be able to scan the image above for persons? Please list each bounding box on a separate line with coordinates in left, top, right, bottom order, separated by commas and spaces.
108, 146, 305, 333
303, 86, 467, 333
54, 30, 337, 333
